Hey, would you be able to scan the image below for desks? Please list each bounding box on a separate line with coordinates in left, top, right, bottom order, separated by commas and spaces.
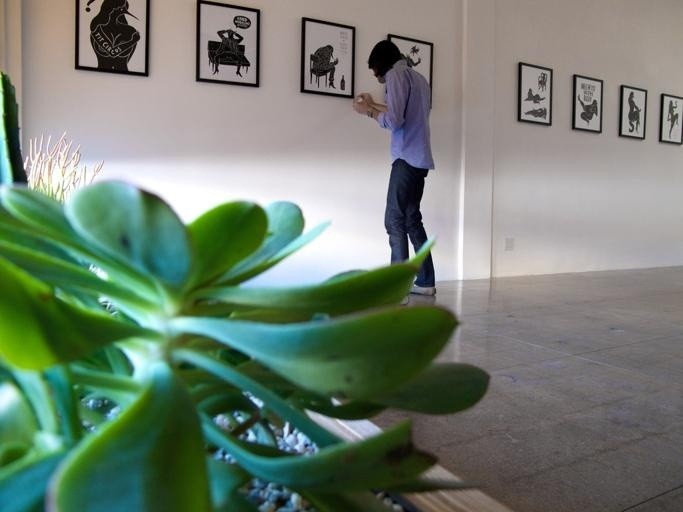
281, 397, 518, 512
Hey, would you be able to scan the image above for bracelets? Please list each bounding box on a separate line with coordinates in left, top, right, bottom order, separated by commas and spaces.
366, 105, 372, 119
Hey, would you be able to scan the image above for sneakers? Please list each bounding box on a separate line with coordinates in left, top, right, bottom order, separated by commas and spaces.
409, 281, 436, 296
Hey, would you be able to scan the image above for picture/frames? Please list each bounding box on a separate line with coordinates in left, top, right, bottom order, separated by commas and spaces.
518, 64, 553, 128
386, 34, 434, 113
572, 74, 604, 133
194, 2, 261, 89
658, 93, 683, 145
300, 17, 357, 99
74, 0, 151, 78
619, 85, 648, 139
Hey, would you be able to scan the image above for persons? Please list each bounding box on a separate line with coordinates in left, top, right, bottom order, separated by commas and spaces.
349, 38, 436, 307
